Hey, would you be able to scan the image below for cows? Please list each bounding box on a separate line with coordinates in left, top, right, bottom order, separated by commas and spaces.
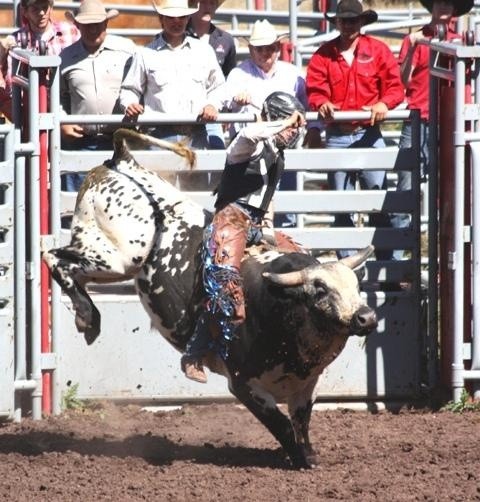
39, 128, 378, 471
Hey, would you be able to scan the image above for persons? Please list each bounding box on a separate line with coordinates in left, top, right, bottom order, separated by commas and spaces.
120, 0, 226, 191
1, 1, 82, 113
303, 0, 406, 292
223, 20, 323, 227
152, 0, 238, 189
181, 90, 309, 383
388, 1, 474, 261
60, 0, 142, 227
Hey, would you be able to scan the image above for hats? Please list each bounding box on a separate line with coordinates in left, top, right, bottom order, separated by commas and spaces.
324, 0, 377, 26
153, 0, 200, 18
418, 0, 475, 18
249, 19, 289, 47
188, 0, 225, 9
65, 0, 120, 25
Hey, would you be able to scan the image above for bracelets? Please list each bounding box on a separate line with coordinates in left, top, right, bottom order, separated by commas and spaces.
311, 127, 320, 133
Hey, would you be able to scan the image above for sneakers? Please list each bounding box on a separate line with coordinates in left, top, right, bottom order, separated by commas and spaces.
181, 354, 208, 384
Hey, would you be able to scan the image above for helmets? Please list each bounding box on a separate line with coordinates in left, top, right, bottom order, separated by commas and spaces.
261, 91, 307, 149
